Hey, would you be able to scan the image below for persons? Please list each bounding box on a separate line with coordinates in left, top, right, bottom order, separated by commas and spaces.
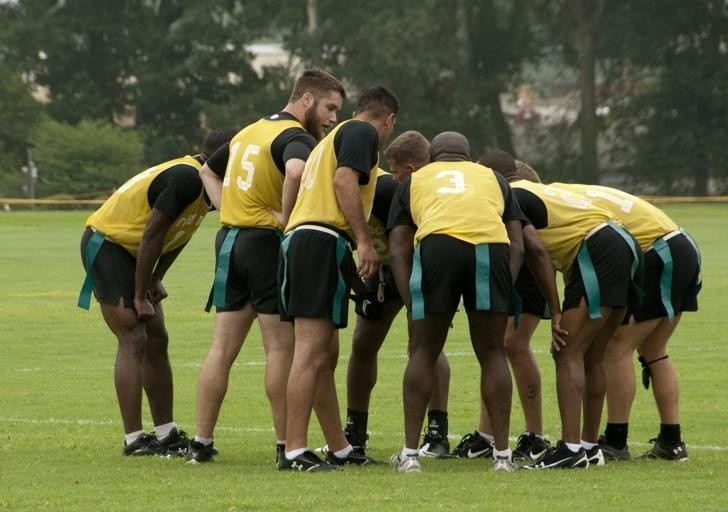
388, 131, 527, 470
182, 67, 347, 470
276, 85, 400, 473
76, 127, 240, 459
517, 159, 701, 462
316, 131, 551, 460
480, 149, 645, 470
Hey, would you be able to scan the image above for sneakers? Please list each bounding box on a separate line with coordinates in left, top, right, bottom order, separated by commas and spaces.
124, 427, 217, 464
275, 430, 388, 474
390, 449, 421, 476
418, 428, 689, 470
